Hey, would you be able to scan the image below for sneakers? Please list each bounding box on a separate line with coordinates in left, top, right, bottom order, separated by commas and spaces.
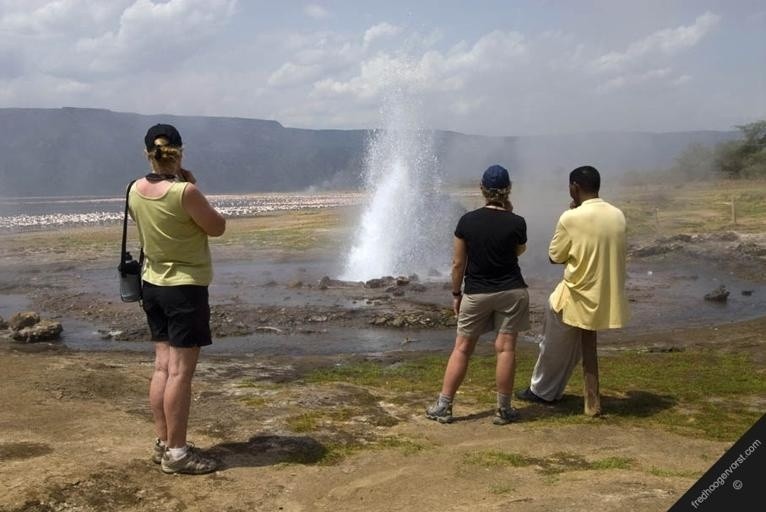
159, 450, 218, 477
514, 387, 548, 404
491, 405, 522, 426
430, 410, 432, 410
424, 397, 455, 424
148, 438, 217, 467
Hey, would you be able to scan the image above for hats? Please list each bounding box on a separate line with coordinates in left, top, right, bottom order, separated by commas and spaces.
480, 164, 511, 190
143, 122, 182, 152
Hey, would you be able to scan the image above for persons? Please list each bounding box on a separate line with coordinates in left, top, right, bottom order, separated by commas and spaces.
125, 123, 225, 474
424, 166, 531, 425
514, 166, 628, 403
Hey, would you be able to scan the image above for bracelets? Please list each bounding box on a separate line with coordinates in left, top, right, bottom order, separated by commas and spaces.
452, 291, 461, 296
